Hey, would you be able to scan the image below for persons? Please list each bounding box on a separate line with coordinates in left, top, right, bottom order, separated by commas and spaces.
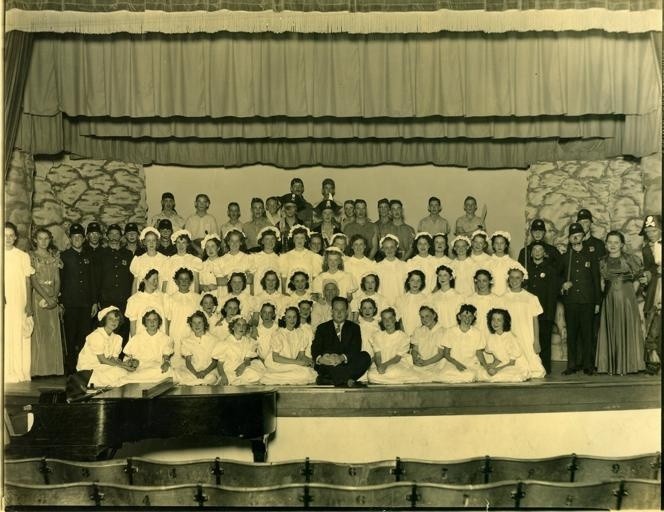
4, 178, 662, 387
